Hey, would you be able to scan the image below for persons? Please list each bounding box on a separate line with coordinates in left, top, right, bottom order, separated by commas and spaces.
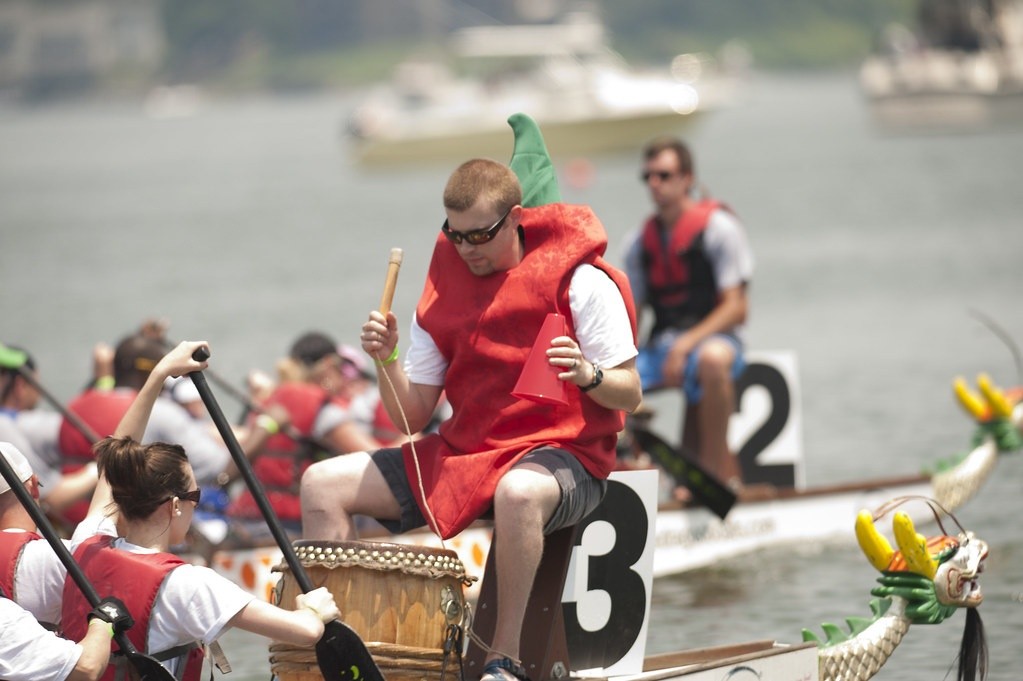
625, 137, 754, 487
59, 340, 340, 681
301, 157, 643, 680
0, 595, 135, 681
18, 322, 421, 551
0, 442, 133, 636
0, 346, 98, 536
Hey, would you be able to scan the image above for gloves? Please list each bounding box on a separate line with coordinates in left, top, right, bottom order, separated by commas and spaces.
87, 596, 134, 636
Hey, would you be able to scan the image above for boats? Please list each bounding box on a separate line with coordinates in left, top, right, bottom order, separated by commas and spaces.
342, 27, 723, 177
571, 494, 991, 680
859, 0, 1023, 135
173, 373, 1023, 602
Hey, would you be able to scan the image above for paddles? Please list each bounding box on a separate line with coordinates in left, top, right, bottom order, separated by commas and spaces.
17, 369, 110, 450
627, 402, 739, 524
202, 365, 270, 415
189, 347, 387, 681
1, 450, 184, 681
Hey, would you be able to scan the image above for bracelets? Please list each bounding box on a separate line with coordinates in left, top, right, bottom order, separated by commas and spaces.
257, 414, 278, 434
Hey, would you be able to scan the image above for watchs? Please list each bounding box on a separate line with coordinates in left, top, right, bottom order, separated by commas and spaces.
578, 363, 603, 393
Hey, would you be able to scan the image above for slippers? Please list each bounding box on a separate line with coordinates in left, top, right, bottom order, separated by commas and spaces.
479, 657, 526, 681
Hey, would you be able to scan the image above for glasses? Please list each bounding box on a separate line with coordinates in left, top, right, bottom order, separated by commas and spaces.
158, 486, 201, 509
442, 206, 512, 245
643, 172, 679, 180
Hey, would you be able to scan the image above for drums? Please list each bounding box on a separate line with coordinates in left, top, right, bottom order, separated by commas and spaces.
267, 537, 479, 679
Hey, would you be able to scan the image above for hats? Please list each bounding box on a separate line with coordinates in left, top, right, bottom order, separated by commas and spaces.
0, 442, 44, 494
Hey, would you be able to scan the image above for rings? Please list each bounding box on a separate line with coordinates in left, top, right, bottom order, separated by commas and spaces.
573, 359, 576, 368
